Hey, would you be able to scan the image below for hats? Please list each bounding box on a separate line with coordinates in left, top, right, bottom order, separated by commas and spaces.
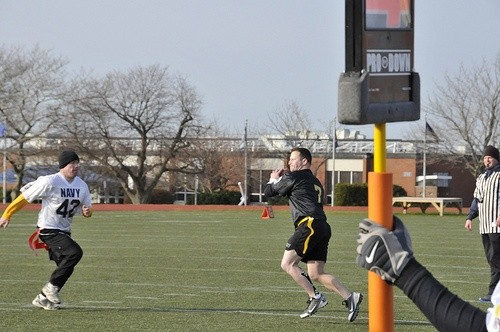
482, 145, 499, 161
59, 151, 79, 169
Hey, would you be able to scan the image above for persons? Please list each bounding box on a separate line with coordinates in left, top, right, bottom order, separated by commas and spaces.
264, 148, 364, 321
356, 215, 500, 332
0, 151, 93, 311
464, 145, 500, 301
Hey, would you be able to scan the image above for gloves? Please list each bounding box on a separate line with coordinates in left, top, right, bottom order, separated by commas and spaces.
356, 215, 414, 285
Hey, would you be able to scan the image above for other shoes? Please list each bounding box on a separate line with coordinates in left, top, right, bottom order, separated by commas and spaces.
479, 294, 492, 302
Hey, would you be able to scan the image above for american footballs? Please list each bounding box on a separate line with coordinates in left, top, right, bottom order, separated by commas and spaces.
278, 169, 290, 177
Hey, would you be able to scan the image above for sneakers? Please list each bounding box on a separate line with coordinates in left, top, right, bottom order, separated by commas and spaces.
32, 293, 59, 310
342, 291, 363, 322
42, 283, 61, 303
300, 293, 328, 319
